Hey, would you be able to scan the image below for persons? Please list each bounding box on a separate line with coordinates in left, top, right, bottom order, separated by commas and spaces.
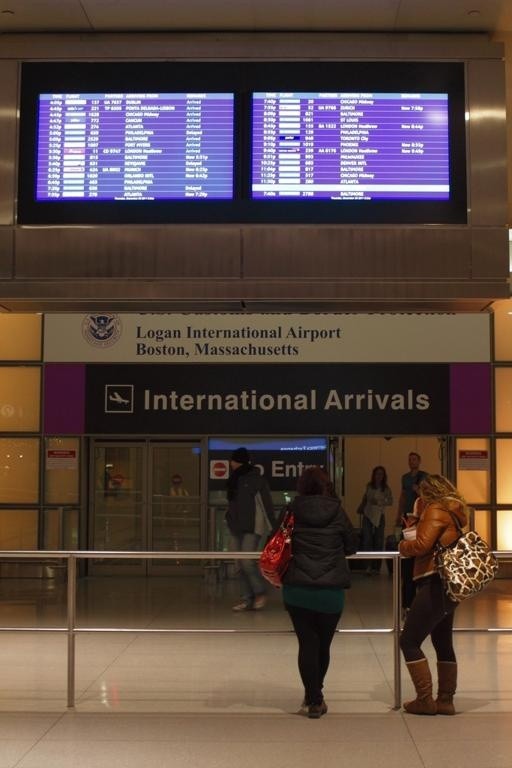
226, 447, 269, 611
395, 451, 466, 715
358, 467, 393, 576
263, 467, 357, 718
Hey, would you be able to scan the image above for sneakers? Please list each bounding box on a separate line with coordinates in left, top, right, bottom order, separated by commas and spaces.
300, 700, 328, 717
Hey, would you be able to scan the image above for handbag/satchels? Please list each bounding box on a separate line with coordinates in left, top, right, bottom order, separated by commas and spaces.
260, 511, 294, 588
437, 532, 499, 600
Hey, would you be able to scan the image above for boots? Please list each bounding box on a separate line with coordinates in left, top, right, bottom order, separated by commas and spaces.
403, 659, 436, 715
433, 661, 456, 715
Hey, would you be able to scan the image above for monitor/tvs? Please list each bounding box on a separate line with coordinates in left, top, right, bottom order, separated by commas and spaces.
16, 60, 239, 224
241, 63, 467, 223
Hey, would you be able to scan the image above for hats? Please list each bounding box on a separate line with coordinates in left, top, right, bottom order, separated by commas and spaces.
232, 448, 249, 463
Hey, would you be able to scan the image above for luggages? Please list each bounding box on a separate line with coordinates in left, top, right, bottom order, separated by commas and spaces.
349, 511, 368, 572
386, 525, 403, 575
